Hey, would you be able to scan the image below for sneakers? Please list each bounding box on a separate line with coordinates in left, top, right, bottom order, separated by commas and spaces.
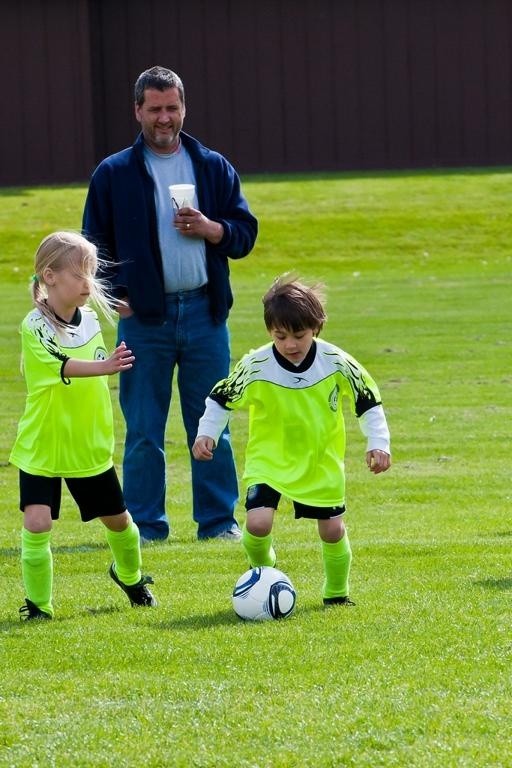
323, 597, 353, 604
20, 600, 52, 619
111, 562, 156, 608
140, 535, 168, 546
218, 527, 243, 543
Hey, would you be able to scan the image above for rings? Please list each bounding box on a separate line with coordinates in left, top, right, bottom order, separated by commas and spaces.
185, 223, 190, 230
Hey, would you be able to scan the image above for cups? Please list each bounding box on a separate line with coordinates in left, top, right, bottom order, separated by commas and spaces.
168, 184, 195, 230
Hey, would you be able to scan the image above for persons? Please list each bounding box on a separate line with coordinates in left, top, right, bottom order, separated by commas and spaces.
8, 229, 156, 622
80, 66, 260, 547
191, 273, 392, 607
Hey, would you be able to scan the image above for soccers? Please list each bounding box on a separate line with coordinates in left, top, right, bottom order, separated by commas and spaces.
232, 566, 296, 620
